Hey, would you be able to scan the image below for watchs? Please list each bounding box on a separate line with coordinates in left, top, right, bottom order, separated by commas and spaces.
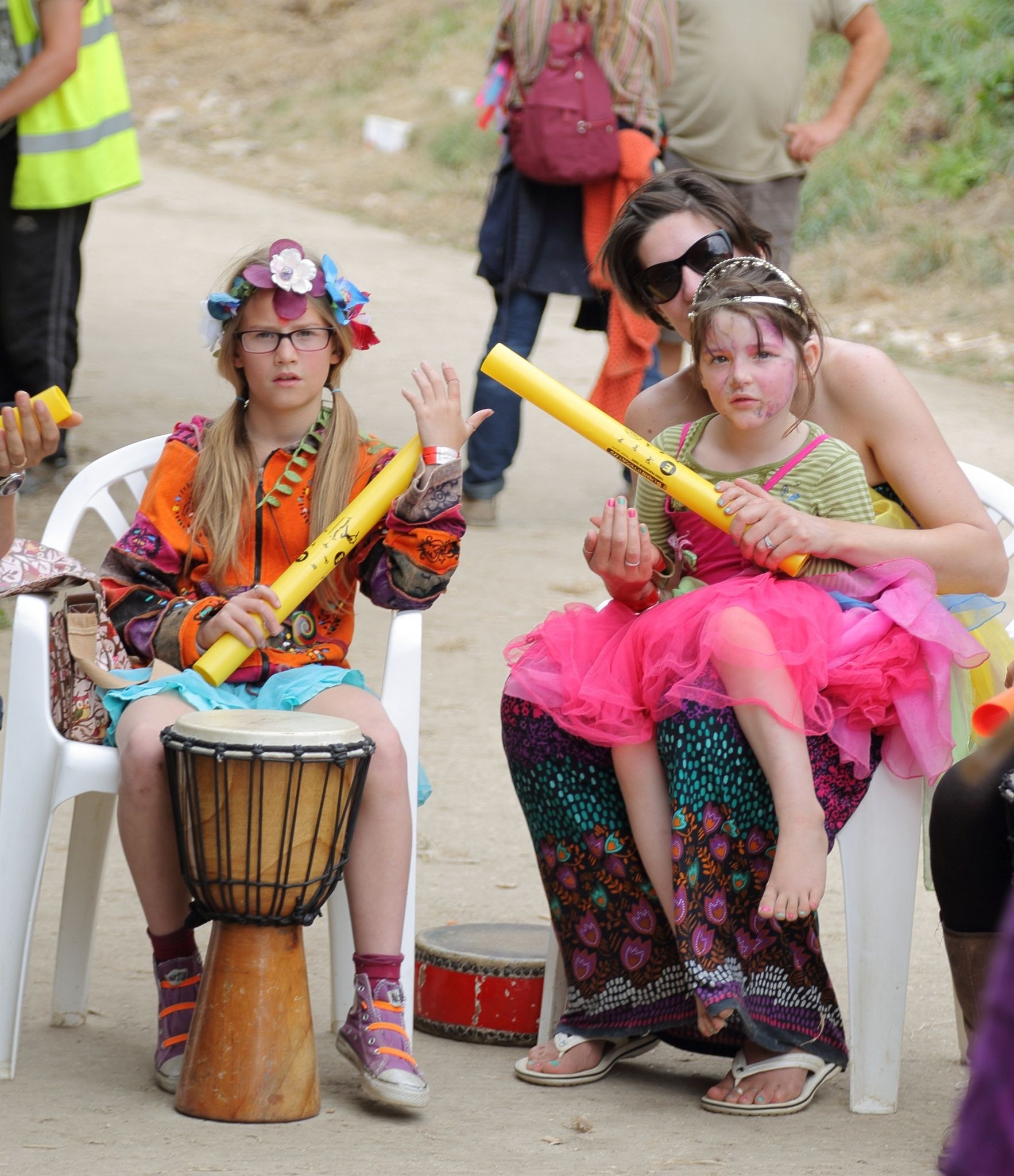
0, 470, 26, 496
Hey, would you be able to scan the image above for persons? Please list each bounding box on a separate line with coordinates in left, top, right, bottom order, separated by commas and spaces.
589, 255, 874, 1036
929, 660, 1014, 1176
0, 0, 142, 468
501, 172, 1009, 1114
96, 241, 494, 1106
0, 391, 84, 560
457, 0, 889, 527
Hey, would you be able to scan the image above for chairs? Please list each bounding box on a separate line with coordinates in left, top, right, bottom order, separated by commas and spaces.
0, 431, 425, 1075
537, 457, 1014, 1116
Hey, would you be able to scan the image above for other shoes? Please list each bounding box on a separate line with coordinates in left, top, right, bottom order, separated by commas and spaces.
459, 495, 497, 527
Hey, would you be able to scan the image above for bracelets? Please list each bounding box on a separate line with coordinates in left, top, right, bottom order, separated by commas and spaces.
422, 446, 458, 465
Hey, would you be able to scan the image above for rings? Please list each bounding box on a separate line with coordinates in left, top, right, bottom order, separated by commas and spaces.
625, 560, 640, 566
582, 542, 594, 554
465, 420, 476, 431
254, 583, 263, 599
10, 457, 27, 468
765, 535, 777, 550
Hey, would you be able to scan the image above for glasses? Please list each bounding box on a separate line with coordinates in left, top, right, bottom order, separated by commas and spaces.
236, 327, 334, 353
635, 229, 738, 305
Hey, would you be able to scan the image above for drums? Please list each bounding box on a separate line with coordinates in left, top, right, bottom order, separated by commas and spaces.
159, 709, 376, 1124
413, 922, 553, 1048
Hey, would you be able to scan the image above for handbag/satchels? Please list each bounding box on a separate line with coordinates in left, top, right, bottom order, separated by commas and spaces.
0, 538, 179, 745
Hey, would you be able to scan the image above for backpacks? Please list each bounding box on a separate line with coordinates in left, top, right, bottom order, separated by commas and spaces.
507, 0, 621, 183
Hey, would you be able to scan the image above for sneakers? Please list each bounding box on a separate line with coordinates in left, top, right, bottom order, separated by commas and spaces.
153, 951, 206, 1093
335, 973, 429, 1107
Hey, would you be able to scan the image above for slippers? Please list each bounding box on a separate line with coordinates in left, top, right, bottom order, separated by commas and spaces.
701, 1049, 842, 1115
513, 1028, 663, 1086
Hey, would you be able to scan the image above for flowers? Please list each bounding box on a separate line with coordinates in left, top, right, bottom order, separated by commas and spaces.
208, 237, 379, 353
